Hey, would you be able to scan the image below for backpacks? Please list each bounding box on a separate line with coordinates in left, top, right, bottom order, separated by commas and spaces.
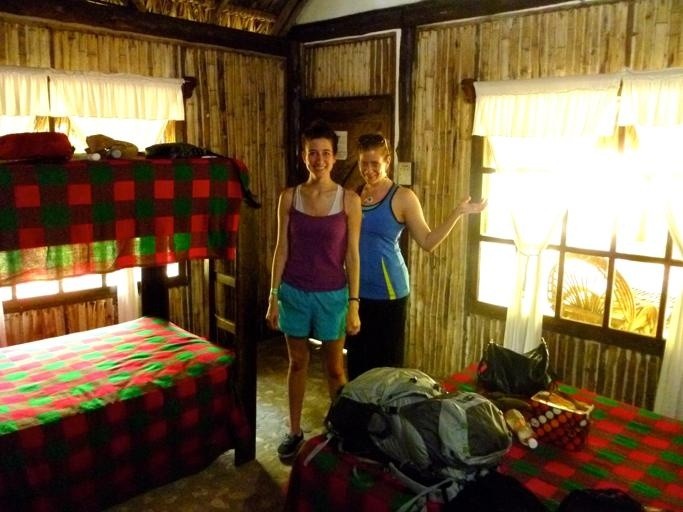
323, 366, 514, 504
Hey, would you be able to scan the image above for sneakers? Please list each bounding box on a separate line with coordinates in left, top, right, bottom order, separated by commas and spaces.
278, 428, 304, 460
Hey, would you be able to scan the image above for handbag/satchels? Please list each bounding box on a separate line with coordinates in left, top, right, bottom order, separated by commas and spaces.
145, 142, 225, 160
475, 336, 559, 400
524, 380, 595, 451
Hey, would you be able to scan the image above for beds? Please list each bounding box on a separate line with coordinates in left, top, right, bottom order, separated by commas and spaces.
0, 148, 261, 284
288, 357, 683, 512
1, 315, 257, 511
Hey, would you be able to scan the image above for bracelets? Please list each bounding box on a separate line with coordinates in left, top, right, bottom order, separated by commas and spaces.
269, 287, 278, 296
347, 298, 360, 302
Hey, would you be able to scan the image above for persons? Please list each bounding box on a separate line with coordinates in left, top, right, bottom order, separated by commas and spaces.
345, 133, 487, 381
264, 127, 363, 460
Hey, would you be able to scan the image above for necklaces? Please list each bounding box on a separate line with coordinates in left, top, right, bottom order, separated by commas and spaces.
364, 188, 376, 204
